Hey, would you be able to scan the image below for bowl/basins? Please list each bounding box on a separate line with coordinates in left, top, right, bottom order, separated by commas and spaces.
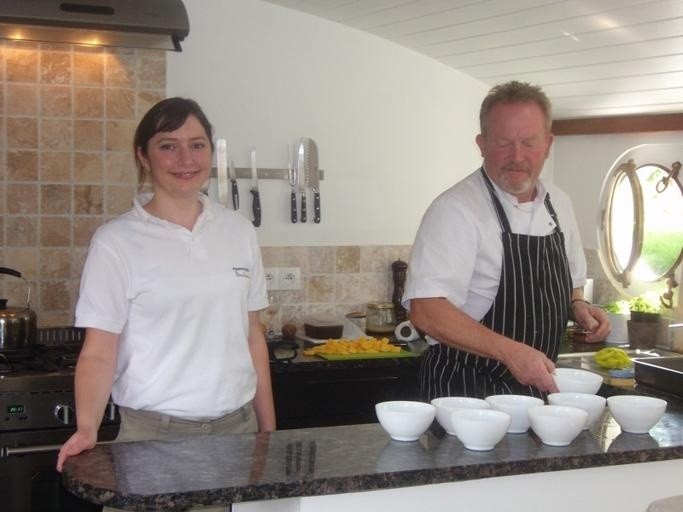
375, 401, 436, 442
449, 410, 510, 451
606, 395, 666, 434
546, 392, 606, 431
549, 367, 602, 395
430, 396, 490, 437
526, 405, 586, 448
484, 394, 543, 435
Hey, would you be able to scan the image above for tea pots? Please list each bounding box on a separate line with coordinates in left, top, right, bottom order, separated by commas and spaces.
0, 266, 37, 354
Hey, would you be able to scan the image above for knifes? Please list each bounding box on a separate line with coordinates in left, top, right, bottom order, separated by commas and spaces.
294, 138, 306, 222
249, 151, 260, 227
307, 139, 320, 224
287, 146, 296, 222
229, 159, 239, 210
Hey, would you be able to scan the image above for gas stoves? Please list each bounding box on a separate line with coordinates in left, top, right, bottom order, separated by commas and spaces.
0, 342, 98, 377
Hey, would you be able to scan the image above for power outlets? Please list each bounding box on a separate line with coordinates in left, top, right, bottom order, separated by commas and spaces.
264, 267, 277, 290
278, 267, 300, 290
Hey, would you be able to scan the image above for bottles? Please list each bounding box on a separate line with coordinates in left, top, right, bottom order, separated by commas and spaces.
364, 300, 395, 333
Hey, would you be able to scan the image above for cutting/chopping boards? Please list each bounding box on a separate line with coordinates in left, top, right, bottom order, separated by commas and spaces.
303, 347, 421, 362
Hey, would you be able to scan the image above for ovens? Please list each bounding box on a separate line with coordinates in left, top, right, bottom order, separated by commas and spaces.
4, 421, 119, 512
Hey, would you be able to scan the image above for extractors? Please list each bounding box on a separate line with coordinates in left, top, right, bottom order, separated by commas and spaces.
0, 0, 190, 51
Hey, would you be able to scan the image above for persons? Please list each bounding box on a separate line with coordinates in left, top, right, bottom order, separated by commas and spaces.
71, 433, 270, 495
55, 98, 279, 475
399, 81, 613, 406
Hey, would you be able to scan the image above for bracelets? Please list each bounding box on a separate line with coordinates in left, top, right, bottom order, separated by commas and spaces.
571, 298, 591, 320
